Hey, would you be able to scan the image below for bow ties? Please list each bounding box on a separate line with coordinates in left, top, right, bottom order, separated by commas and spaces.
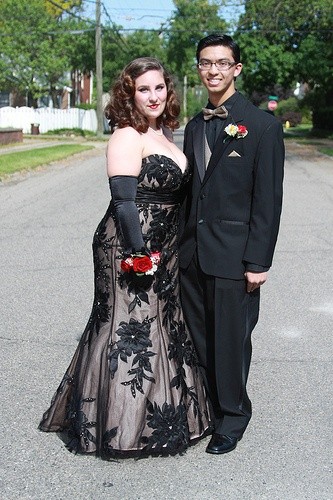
201, 106, 228, 121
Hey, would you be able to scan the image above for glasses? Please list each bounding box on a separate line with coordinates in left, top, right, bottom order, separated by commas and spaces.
198, 60, 235, 70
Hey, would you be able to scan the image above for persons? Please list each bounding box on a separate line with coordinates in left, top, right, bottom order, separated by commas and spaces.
167, 33, 284, 455
37, 56, 215, 464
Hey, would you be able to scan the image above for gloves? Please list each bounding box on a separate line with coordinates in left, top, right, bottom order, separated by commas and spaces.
109, 175, 149, 257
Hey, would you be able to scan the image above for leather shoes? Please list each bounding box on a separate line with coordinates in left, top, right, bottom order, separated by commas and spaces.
206, 433, 242, 453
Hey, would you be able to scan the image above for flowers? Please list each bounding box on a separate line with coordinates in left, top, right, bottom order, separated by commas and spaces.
222, 124, 249, 143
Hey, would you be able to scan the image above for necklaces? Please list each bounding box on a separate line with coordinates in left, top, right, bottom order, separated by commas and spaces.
148, 124, 164, 136
